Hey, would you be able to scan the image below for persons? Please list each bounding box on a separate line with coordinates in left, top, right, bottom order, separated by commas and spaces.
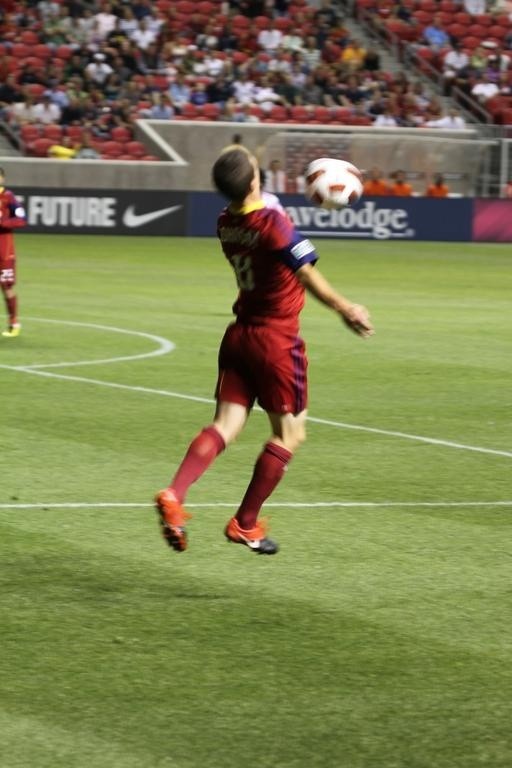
0, 162, 29, 337
155, 143, 374, 557
0, 0, 512, 158
215, 133, 450, 199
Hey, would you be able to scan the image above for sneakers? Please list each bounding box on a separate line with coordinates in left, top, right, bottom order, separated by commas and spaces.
2, 322, 23, 338
156, 484, 193, 553
224, 517, 282, 555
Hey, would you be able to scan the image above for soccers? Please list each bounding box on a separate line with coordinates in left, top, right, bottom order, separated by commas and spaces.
305, 158, 363, 209
